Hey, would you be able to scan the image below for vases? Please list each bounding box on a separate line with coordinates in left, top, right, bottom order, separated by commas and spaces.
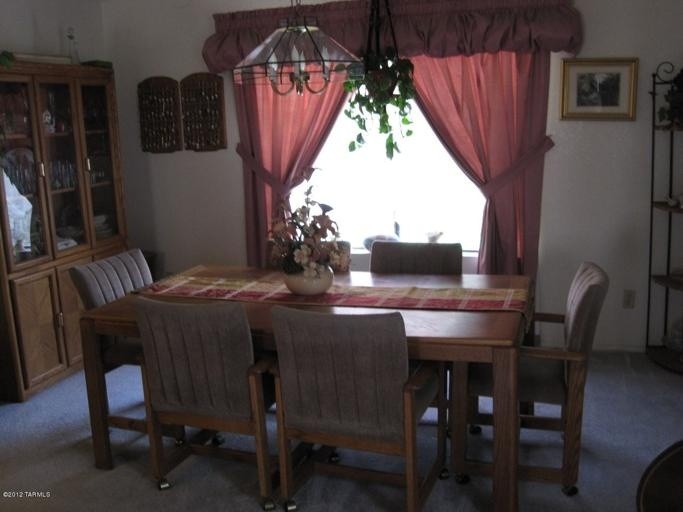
284, 264, 334, 294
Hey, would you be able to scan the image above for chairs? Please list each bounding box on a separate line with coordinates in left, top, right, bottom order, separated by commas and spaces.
68, 248, 186, 445
369, 240, 462, 437
270, 304, 449, 512
133, 294, 313, 511
264, 238, 351, 274
450, 259, 610, 496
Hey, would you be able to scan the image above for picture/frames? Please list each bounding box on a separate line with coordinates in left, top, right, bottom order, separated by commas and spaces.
559, 56, 640, 122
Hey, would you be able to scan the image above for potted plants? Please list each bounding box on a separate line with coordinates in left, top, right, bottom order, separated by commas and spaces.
338, 46, 417, 160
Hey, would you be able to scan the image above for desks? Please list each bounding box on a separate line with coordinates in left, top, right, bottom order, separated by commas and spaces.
77, 261, 536, 512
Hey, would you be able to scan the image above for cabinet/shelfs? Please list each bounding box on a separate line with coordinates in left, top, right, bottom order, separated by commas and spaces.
0, 58, 129, 402
645, 61, 683, 378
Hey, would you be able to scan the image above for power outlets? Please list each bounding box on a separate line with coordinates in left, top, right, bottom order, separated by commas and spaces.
623, 290, 636, 310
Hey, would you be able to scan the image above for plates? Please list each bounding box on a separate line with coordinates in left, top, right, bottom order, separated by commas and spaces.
2, 147, 34, 176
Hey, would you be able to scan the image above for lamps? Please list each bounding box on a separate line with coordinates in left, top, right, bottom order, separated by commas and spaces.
231, 0, 367, 96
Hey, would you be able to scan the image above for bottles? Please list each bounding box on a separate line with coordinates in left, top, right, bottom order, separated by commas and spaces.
10, 157, 105, 193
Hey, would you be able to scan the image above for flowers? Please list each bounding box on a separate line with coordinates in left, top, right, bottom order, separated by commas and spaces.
266, 165, 340, 275
